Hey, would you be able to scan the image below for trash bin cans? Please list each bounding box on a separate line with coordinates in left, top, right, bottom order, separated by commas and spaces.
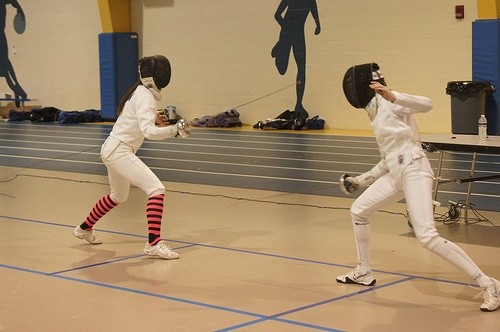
448, 81, 485, 135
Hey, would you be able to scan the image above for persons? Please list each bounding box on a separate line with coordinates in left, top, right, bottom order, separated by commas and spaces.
74, 55, 179, 259
336, 62, 500, 311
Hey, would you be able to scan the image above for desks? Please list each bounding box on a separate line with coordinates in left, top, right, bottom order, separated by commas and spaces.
420, 132, 500, 225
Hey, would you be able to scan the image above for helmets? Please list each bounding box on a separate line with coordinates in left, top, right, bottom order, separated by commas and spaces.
342, 63, 386, 109
138, 55, 171, 88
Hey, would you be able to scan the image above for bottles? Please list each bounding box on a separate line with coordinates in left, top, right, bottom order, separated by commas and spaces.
478, 114, 487, 141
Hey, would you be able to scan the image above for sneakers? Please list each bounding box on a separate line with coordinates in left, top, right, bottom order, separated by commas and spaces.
336, 265, 376, 286
143, 240, 180, 259
72, 226, 103, 244
468, 276, 500, 312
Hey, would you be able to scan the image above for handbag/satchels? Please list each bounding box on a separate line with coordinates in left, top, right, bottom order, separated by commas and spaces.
306, 115, 325, 129
191, 110, 243, 127
9, 107, 62, 122
58, 109, 101, 123
253, 110, 295, 129
